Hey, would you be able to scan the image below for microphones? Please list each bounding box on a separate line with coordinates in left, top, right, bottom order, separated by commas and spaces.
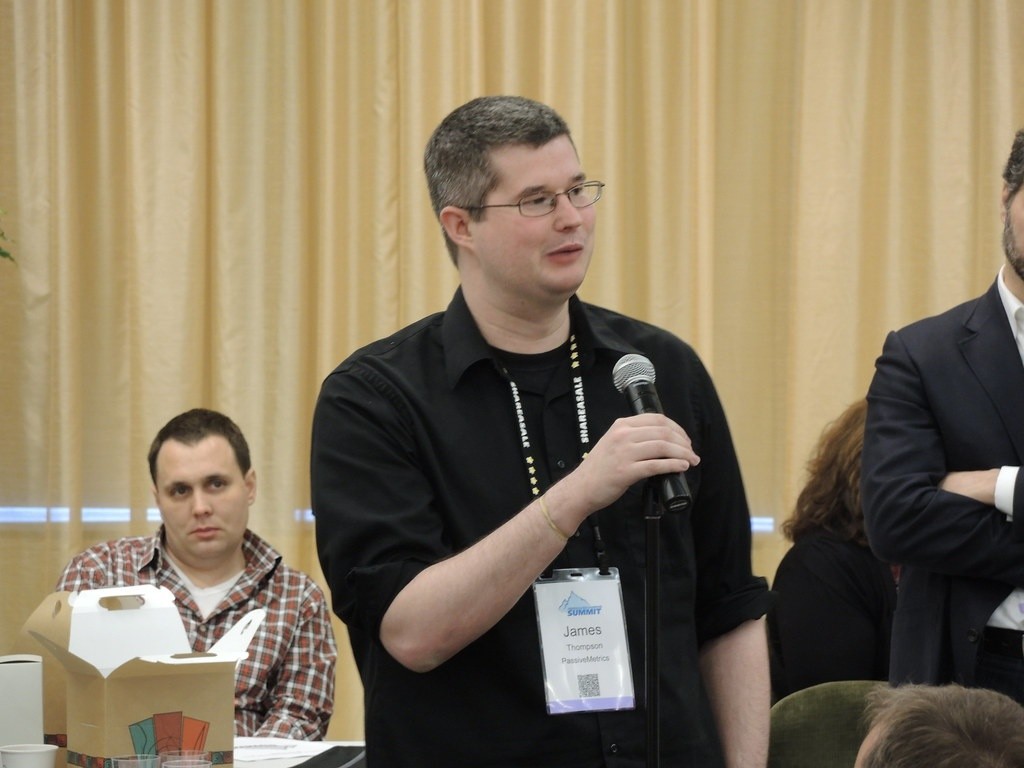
612, 353, 692, 511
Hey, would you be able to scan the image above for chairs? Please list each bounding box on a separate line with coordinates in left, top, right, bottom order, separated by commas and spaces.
768, 679, 888, 768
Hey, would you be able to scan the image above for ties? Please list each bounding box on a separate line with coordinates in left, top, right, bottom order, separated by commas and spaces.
1015, 306, 1024, 368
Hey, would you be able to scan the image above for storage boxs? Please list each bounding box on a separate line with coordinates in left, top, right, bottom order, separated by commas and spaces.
20, 585, 268, 768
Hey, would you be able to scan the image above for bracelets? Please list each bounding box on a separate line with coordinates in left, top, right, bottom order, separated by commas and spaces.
540, 496, 569, 541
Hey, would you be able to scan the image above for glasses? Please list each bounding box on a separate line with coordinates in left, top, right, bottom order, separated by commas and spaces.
462, 180, 606, 218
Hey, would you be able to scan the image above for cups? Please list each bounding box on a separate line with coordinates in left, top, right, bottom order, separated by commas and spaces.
112, 754, 160, 768
163, 760, 213, 768
161, 749, 207, 760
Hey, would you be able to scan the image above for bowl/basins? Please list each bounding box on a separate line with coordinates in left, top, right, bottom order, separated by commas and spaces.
0, 744, 59, 768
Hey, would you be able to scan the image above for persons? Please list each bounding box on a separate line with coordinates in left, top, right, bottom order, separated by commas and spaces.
309, 95, 769, 768
56, 408, 338, 740
864, 128, 1024, 705
766, 398, 900, 707
854, 682, 1024, 768
